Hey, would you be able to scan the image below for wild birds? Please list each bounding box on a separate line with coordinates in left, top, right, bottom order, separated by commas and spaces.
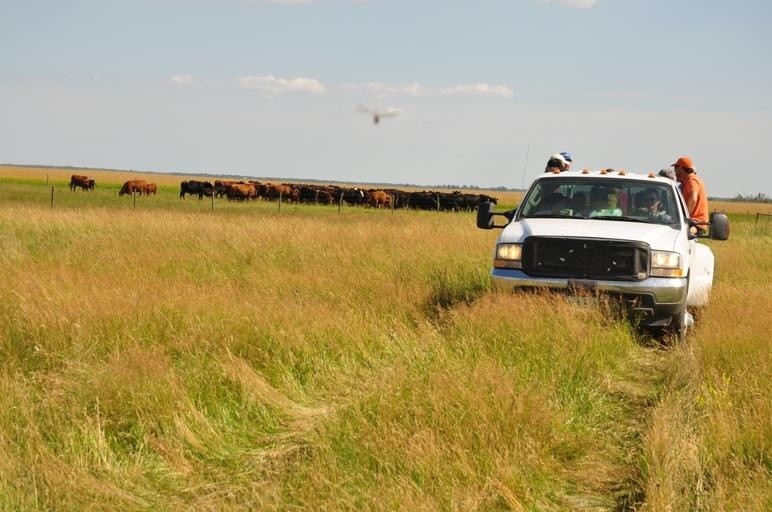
360, 105, 402, 123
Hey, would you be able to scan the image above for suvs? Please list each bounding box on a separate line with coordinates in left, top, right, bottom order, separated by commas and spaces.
474, 168, 732, 350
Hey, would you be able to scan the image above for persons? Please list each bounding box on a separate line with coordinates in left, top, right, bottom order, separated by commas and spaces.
546, 153, 570, 174
670, 157, 708, 232
560, 152, 572, 172
659, 168, 675, 180
632, 188, 671, 221
675, 166, 696, 194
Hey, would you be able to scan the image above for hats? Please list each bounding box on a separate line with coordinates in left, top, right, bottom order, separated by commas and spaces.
670, 157, 694, 170
560, 152, 573, 161
550, 154, 570, 165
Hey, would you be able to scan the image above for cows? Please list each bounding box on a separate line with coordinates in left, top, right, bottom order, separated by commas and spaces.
146, 183, 157, 196
180, 181, 499, 213
70, 175, 89, 193
119, 180, 147, 198
88, 180, 95, 192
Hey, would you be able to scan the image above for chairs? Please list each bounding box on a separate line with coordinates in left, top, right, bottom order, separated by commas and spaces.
539, 190, 667, 219
